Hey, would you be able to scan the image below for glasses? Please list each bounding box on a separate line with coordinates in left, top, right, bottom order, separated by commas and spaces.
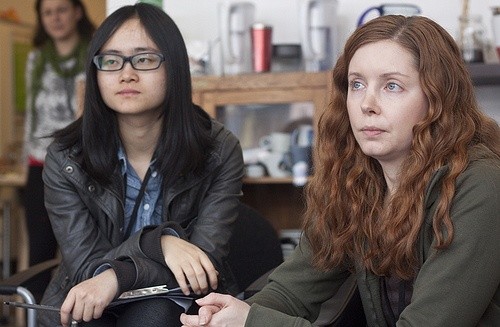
92, 52, 164, 72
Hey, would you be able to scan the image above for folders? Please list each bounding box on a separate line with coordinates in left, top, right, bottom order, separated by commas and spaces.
5, 283, 196, 315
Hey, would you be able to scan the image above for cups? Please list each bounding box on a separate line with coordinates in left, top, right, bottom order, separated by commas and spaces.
249, 26, 273, 73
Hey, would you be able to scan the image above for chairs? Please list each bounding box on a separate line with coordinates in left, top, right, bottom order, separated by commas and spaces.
0, 203, 283, 327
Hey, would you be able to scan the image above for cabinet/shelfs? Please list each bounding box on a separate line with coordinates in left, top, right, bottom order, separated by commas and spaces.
190, 70, 333, 231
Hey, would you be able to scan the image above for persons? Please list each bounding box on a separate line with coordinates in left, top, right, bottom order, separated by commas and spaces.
18, 0, 97, 327
42, 3, 245, 327
180, 15, 500, 327
